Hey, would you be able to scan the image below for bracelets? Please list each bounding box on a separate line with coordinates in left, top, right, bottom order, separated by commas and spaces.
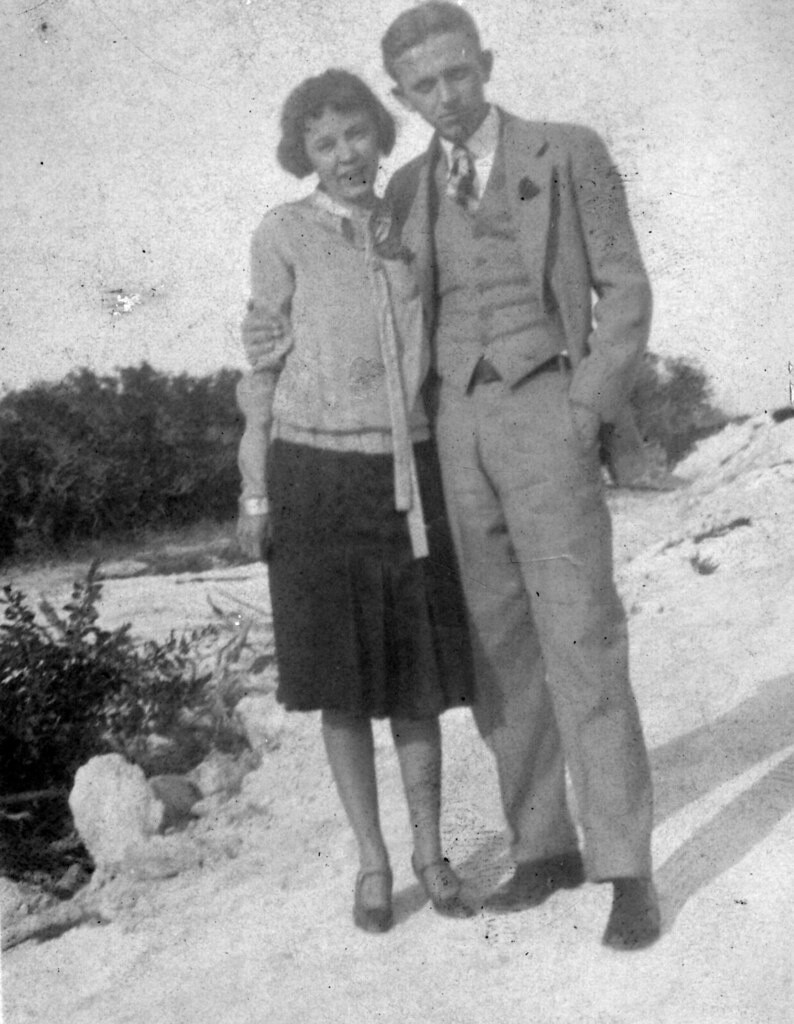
244, 498, 269, 516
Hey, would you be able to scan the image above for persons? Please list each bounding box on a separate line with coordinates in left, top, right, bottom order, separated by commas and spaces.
234, 64, 486, 936
241, 0, 661, 951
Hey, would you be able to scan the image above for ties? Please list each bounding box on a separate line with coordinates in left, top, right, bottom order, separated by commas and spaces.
447, 144, 476, 209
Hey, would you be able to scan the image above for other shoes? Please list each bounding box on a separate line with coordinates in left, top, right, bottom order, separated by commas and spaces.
411, 852, 476, 919
600, 875, 660, 951
483, 850, 586, 914
353, 866, 393, 932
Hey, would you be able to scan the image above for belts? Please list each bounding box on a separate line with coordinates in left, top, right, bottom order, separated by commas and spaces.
474, 354, 572, 384
